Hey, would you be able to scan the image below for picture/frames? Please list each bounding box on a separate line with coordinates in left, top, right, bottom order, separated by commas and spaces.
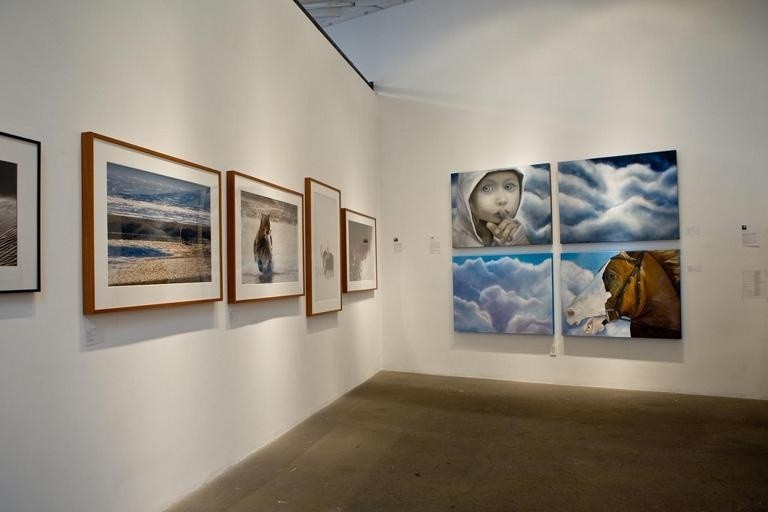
303, 177, 345, 317
0, 129, 43, 293
341, 208, 381, 295
224, 169, 306, 306
80, 130, 223, 314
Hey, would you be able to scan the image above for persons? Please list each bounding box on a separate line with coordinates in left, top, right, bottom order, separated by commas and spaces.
452, 167, 529, 247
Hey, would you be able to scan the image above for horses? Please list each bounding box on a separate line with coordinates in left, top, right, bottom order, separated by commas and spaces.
562, 249, 681, 339
